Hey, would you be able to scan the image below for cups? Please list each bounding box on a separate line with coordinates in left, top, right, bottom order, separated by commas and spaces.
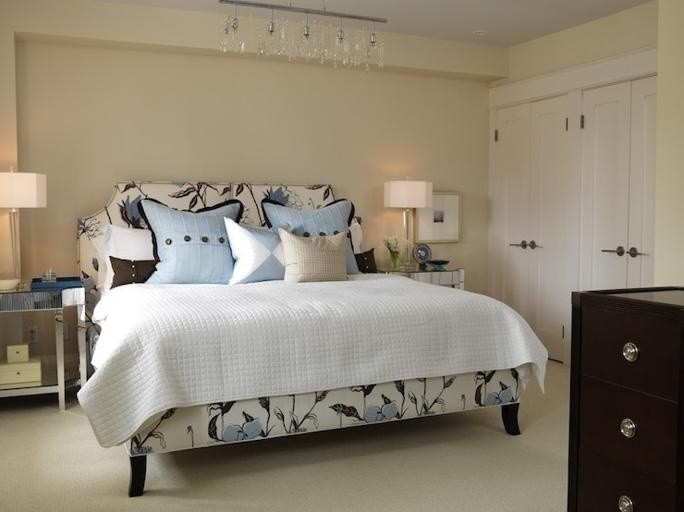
41, 270, 56, 283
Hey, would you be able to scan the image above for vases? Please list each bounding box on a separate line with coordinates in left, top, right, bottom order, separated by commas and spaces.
390, 251, 399, 267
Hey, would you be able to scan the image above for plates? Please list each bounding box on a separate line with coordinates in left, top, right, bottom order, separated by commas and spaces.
427, 260, 450, 266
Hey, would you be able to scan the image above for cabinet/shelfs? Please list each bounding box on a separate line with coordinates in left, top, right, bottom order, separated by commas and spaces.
579, 72, 656, 290
489, 92, 571, 364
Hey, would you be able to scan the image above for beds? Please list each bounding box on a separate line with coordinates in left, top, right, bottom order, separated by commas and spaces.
76, 180, 549, 498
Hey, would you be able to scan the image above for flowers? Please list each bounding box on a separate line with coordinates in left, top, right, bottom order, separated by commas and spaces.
382, 233, 411, 252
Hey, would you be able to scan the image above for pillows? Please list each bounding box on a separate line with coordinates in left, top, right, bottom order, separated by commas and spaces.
92, 223, 155, 290
137, 198, 244, 285
278, 227, 348, 283
354, 248, 377, 273
223, 216, 284, 285
261, 198, 359, 275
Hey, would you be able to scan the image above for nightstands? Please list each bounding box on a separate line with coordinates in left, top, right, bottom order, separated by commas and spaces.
0, 278, 95, 411
377, 262, 464, 290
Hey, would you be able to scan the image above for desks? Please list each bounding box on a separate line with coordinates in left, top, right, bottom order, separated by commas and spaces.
567, 286, 684, 512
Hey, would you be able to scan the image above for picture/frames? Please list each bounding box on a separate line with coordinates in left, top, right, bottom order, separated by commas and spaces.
413, 191, 463, 244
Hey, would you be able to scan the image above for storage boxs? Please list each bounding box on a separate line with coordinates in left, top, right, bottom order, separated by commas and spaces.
0, 356, 43, 389
7, 344, 29, 363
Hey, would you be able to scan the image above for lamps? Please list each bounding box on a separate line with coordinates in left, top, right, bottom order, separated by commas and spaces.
218, 0, 386, 71
383, 176, 432, 271
0, 172, 48, 291
